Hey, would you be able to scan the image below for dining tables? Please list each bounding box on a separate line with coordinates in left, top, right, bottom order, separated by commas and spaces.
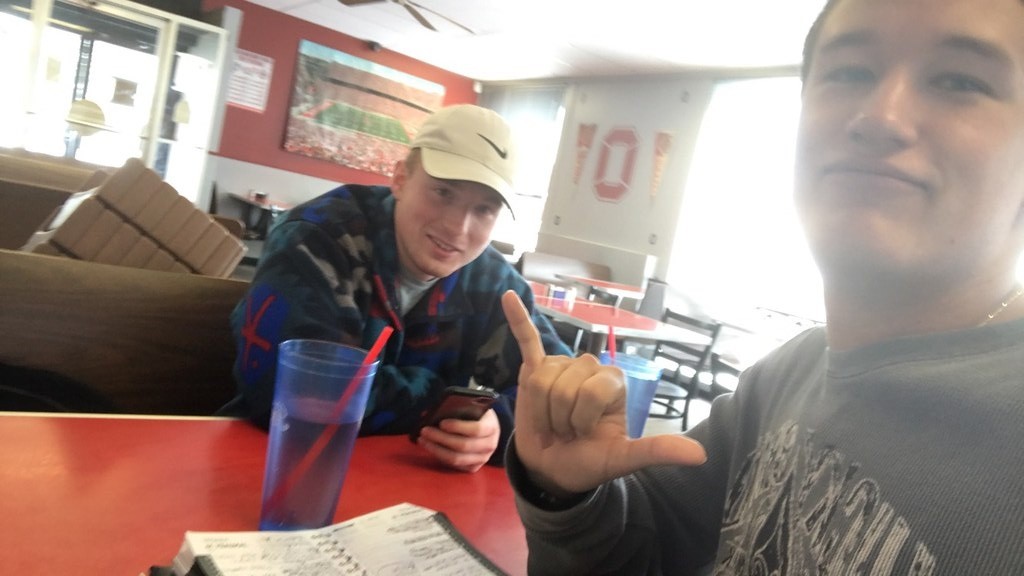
558, 276, 643, 354
2, 412, 527, 576
534, 296, 712, 359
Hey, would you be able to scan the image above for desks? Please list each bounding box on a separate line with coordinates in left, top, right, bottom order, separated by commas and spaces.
229, 192, 287, 236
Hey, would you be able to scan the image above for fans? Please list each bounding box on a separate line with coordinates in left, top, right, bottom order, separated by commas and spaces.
338, 0, 477, 37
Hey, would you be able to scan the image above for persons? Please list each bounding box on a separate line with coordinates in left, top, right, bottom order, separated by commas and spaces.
500, 0, 1023, 576
213, 103, 579, 476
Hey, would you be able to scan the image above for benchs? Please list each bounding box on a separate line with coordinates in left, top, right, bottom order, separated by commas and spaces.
0, 154, 108, 250
0, 252, 252, 417
521, 252, 623, 351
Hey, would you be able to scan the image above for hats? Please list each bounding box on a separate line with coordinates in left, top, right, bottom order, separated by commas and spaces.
410, 103, 517, 222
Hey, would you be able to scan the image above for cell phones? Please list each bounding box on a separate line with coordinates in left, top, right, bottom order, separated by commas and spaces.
410, 386, 494, 444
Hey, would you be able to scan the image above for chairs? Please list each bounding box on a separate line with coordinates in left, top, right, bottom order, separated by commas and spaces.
647, 309, 718, 431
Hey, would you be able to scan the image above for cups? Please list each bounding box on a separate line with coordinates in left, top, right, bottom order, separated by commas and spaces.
598, 350, 663, 441
258, 339, 380, 532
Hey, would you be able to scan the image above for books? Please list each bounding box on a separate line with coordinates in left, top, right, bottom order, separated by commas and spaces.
145, 501, 509, 576
